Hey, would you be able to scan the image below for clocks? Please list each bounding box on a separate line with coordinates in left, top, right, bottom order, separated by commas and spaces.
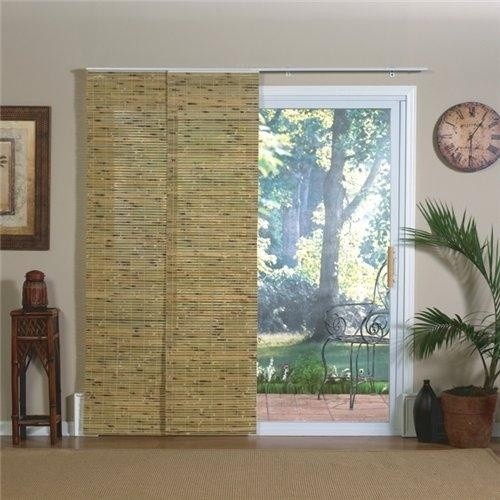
433, 102, 500, 172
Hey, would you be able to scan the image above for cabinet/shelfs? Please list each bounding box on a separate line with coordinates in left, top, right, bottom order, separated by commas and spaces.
9, 308, 62, 447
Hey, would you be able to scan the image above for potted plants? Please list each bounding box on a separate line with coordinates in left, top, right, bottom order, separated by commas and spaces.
397, 197, 500, 447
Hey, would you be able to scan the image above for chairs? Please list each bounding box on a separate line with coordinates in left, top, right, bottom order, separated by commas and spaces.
317, 259, 390, 410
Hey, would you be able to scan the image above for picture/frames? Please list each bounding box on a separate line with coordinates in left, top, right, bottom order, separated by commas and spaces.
0, 105, 52, 250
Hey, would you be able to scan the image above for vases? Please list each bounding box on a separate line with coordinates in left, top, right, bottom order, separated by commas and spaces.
413, 379, 441, 443
